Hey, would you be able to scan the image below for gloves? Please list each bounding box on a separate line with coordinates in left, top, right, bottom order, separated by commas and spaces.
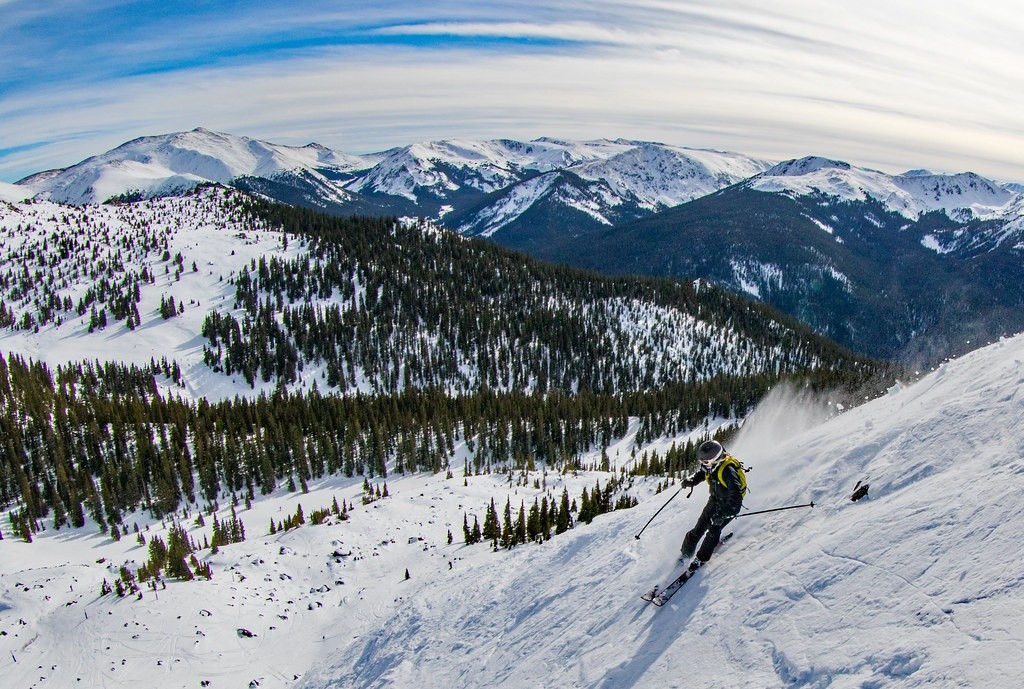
681, 478, 694, 489
713, 514, 724, 527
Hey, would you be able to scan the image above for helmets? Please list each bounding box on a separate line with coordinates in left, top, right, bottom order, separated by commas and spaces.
697, 440, 723, 460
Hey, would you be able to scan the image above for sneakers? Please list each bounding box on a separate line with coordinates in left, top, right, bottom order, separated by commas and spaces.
689, 558, 707, 570
676, 554, 687, 564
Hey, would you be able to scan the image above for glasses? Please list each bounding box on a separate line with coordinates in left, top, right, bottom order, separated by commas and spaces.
698, 460, 711, 466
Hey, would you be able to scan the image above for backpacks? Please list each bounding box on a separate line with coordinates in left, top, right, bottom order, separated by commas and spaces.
704, 455, 747, 500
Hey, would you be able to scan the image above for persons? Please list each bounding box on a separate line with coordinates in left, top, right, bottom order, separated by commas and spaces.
675, 441, 743, 570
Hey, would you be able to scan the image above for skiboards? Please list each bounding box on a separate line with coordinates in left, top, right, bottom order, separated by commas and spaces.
639, 532, 733, 607
